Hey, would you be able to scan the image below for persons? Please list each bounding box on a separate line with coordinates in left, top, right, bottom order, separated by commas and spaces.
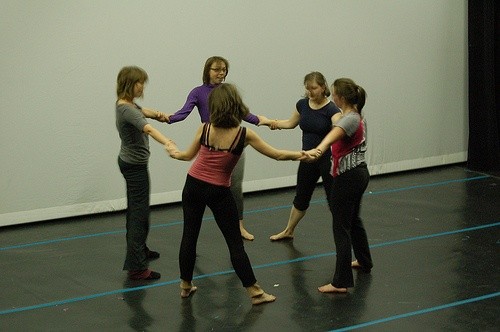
156, 57, 282, 241
114, 65, 181, 281
293, 77, 373, 293
256, 71, 343, 242
164, 82, 323, 305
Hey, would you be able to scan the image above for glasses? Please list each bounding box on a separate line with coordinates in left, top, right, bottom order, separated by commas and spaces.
209, 66, 227, 72
